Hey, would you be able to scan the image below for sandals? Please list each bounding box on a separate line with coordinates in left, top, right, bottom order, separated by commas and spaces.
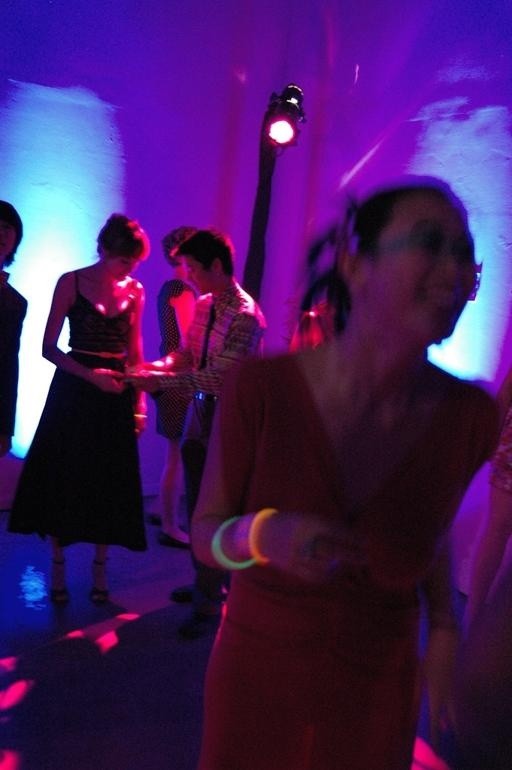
90, 556, 109, 605
50, 559, 68, 605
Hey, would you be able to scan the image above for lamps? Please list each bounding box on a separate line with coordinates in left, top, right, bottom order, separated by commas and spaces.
263, 82, 306, 149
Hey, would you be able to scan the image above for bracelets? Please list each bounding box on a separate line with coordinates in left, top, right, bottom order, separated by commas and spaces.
248, 507, 279, 567
210, 516, 258, 572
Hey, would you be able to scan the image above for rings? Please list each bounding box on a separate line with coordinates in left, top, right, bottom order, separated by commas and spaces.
301, 538, 321, 564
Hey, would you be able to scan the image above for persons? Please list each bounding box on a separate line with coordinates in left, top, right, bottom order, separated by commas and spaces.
285, 271, 350, 354
2, 212, 153, 604
1, 200, 31, 470
118, 227, 268, 644
184, 170, 504, 768
146, 223, 205, 549
458, 347, 512, 632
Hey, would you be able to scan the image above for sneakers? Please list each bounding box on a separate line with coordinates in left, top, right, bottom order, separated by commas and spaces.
176, 604, 219, 640
170, 581, 214, 602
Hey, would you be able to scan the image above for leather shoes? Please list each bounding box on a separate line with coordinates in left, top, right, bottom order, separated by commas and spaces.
144, 507, 193, 548
